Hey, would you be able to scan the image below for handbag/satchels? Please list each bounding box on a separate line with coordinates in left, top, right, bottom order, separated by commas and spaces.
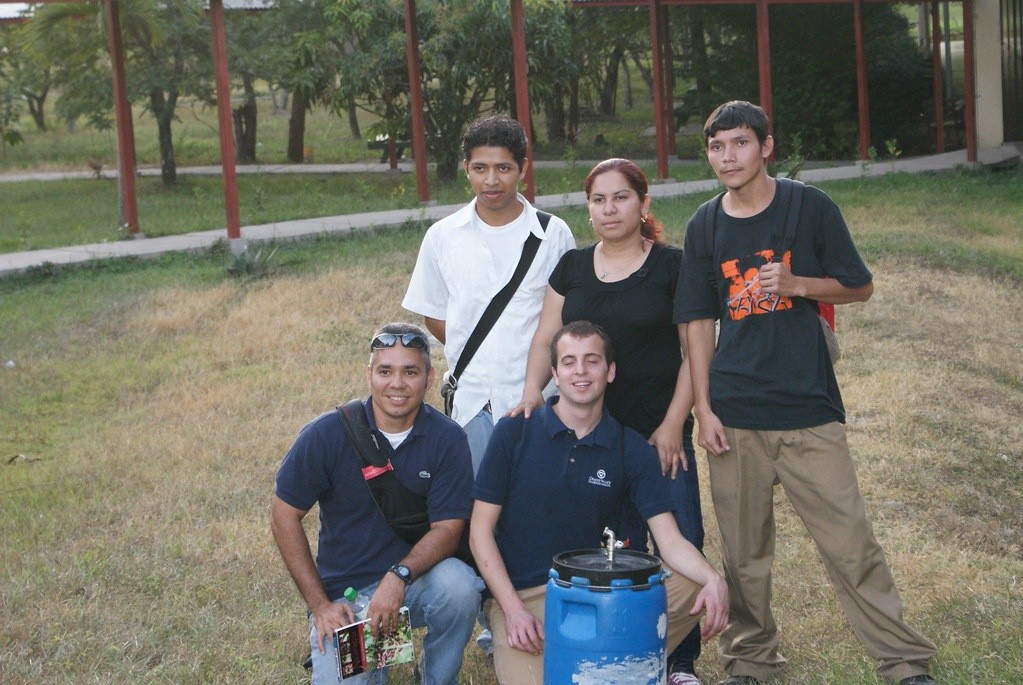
392, 504, 492, 598
440, 383, 456, 416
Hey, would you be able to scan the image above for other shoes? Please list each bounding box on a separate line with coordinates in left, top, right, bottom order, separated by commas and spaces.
303, 656, 313, 673
476, 628, 494, 659
412, 663, 424, 685
899, 674, 936, 685
718, 675, 760, 685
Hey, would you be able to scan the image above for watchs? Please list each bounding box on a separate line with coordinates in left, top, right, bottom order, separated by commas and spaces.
388, 564, 413, 584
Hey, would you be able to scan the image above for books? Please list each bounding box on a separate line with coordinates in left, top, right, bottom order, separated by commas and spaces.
335, 606, 415, 680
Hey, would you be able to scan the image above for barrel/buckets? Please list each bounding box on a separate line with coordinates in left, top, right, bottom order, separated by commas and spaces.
542, 550, 669, 685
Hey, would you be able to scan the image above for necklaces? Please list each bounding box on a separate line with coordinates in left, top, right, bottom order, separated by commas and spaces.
598, 251, 643, 280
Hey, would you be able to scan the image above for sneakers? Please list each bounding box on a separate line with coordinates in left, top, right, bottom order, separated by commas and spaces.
666, 671, 702, 685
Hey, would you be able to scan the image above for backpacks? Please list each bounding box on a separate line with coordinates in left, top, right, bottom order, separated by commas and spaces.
693, 178, 840, 365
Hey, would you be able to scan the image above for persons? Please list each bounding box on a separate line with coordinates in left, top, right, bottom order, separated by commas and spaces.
680, 100, 940, 685
500, 152, 706, 685
468, 320, 730, 685
401, 111, 577, 660
268, 323, 483, 685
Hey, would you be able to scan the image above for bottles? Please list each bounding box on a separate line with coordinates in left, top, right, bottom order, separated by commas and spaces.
344, 587, 371, 621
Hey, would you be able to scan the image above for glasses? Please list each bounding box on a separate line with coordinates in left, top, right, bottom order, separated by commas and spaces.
370, 333, 430, 357
